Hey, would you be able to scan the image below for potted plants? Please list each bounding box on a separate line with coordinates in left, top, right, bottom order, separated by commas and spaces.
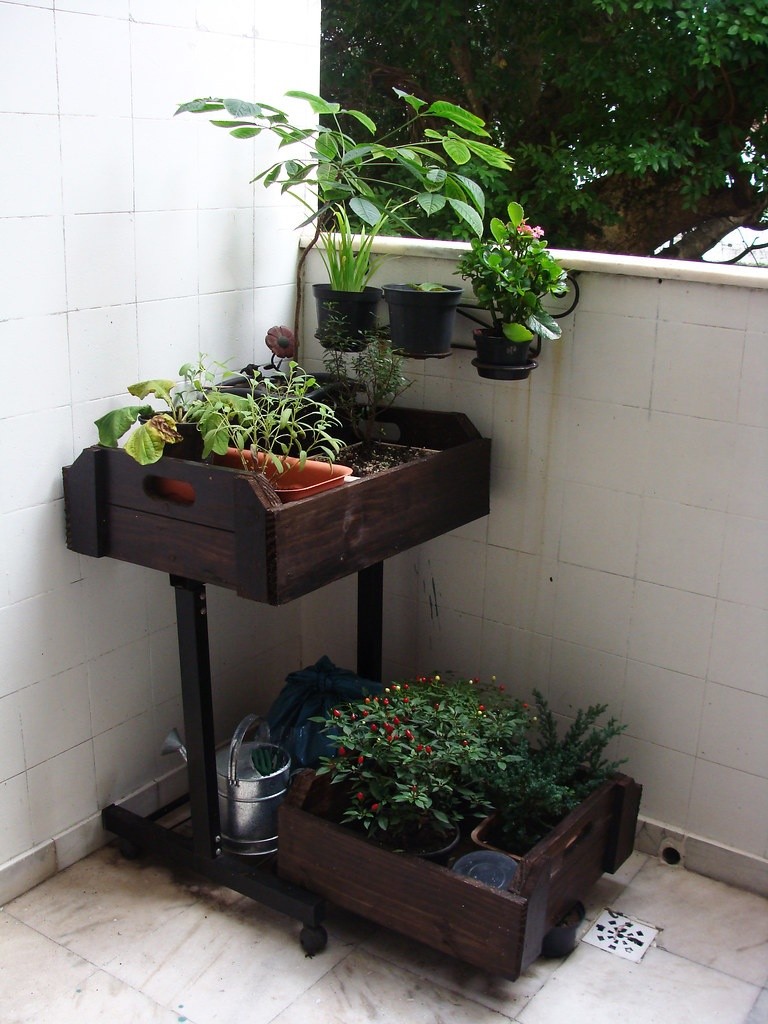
308, 667, 630, 869
93, 301, 444, 501
380, 282, 466, 354
285, 187, 419, 353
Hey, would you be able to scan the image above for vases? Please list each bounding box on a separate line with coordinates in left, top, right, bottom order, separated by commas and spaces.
217, 371, 367, 419
472, 327, 532, 365
540, 900, 587, 956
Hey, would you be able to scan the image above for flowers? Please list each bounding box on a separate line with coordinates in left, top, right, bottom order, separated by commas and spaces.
264, 326, 299, 381
452, 202, 574, 343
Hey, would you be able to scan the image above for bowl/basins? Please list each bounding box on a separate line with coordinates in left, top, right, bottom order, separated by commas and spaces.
452, 851, 519, 893
541, 899, 586, 958
